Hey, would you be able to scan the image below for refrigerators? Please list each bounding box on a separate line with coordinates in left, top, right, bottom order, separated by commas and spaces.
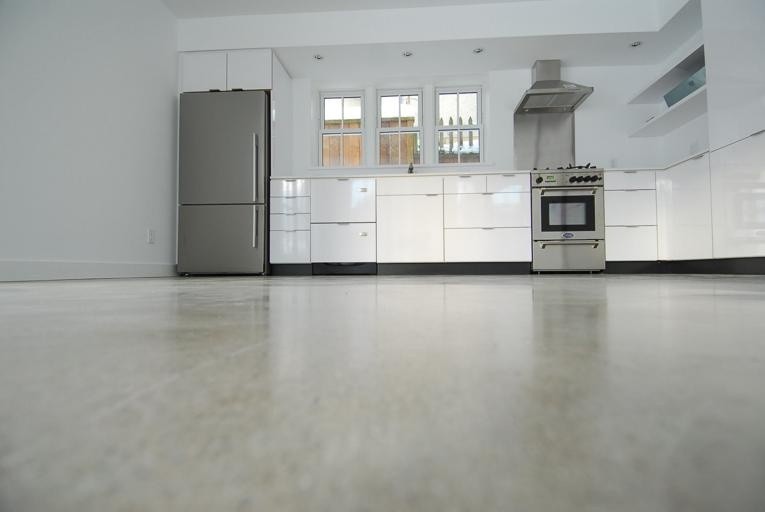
175, 92, 268, 278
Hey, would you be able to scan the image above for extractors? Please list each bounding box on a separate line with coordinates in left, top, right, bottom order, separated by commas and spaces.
513, 59, 593, 116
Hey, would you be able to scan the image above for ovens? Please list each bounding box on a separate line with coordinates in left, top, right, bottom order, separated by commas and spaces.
531, 187, 605, 274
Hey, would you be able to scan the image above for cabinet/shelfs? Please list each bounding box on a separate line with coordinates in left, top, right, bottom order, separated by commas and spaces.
712, 129, 765, 258
603, 169, 658, 262
622, 44, 708, 140
375, 173, 532, 265
179, 49, 273, 92
267, 176, 376, 264
657, 147, 712, 263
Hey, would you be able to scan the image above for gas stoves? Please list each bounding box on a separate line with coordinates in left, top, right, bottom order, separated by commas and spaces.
531, 163, 604, 187
